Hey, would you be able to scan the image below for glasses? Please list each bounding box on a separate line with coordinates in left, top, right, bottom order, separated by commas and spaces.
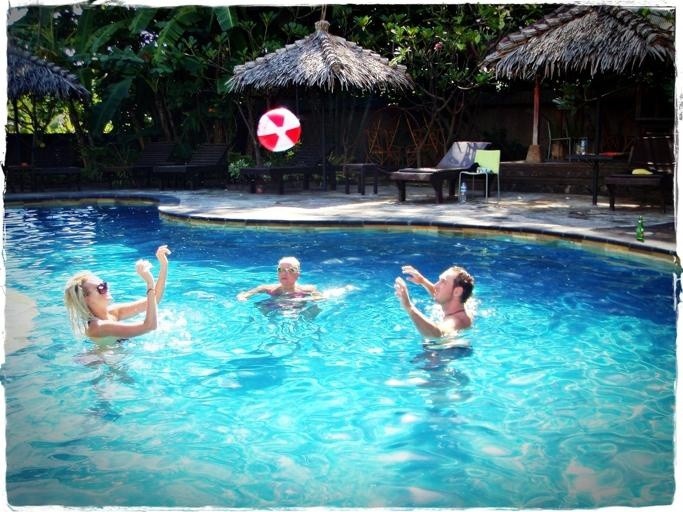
96, 282, 108, 295
277, 267, 298, 273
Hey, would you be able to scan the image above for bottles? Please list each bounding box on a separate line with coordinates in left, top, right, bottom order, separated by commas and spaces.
460, 182, 467, 203
635, 215, 644, 243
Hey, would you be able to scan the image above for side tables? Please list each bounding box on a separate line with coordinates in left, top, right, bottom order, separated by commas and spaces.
342, 163, 379, 194
564, 153, 628, 205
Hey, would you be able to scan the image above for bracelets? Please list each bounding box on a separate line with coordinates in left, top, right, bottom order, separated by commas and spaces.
145, 288, 156, 297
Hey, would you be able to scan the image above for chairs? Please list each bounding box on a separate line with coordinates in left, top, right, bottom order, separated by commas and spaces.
239, 142, 339, 194
604, 134, 673, 215
7, 135, 81, 193
388, 140, 501, 204
100, 140, 232, 190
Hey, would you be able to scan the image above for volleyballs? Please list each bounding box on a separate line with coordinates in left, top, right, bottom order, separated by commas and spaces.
256, 108, 303, 153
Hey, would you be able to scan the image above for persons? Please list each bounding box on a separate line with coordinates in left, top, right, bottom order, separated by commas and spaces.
63, 244, 171, 351
234, 255, 328, 306
394, 262, 478, 341
